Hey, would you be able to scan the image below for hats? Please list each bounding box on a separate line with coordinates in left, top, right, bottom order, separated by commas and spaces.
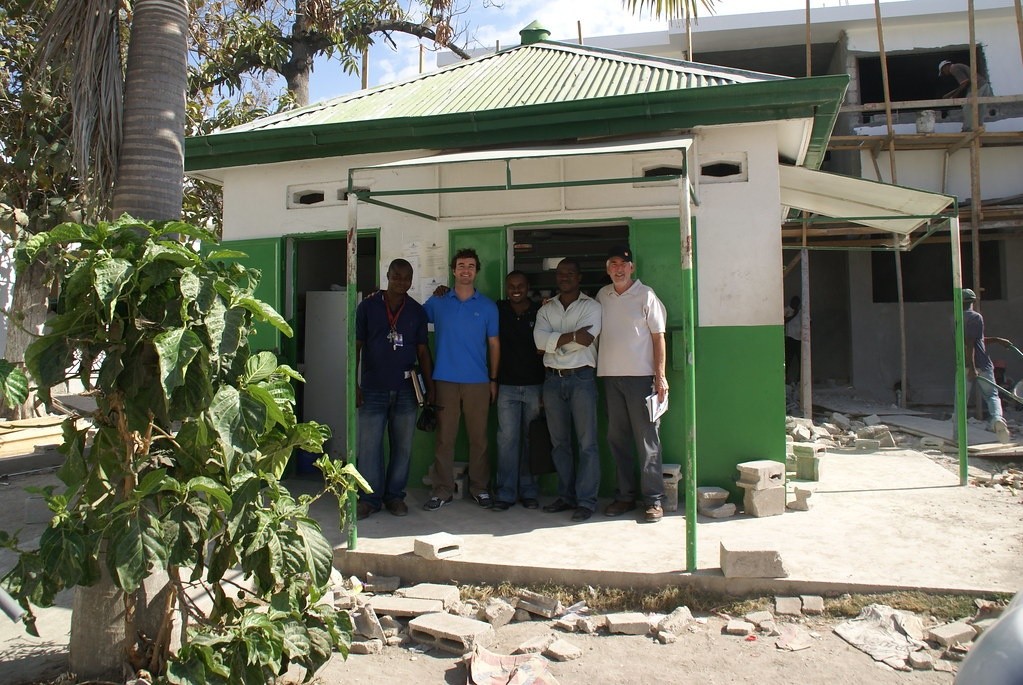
606, 247, 634, 262
963, 289, 976, 303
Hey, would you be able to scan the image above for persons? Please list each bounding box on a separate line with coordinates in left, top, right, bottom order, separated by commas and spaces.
432, 272, 545, 511
542, 246, 669, 522
356, 259, 437, 522
937, 60, 990, 132
532, 258, 601, 522
420, 248, 500, 511
784, 296, 813, 385
952, 288, 1010, 444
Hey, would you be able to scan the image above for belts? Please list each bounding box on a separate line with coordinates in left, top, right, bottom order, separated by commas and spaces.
549, 366, 589, 378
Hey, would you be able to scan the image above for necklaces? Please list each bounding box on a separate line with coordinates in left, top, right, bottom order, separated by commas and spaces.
384, 291, 404, 342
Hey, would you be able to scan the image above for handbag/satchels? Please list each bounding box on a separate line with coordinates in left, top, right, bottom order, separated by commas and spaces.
416, 403, 442, 432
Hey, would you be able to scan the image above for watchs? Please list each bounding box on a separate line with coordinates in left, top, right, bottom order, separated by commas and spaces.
489, 377, 499, 382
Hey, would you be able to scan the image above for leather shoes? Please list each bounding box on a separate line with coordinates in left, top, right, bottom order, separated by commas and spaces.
604, 499, 638, 517
642, 505, 663, 522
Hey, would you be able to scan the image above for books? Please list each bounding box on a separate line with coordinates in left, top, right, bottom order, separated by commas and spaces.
645, 391, 669, 422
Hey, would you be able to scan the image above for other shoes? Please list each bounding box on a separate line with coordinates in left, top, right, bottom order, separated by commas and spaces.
571, 506, 591, 522
386, 499, 409, 516
492, 500, 515, 511
523, 498, 539, 509
355, 502, 382, 520
995, 422, 1011, 443
543, 499, 574, 513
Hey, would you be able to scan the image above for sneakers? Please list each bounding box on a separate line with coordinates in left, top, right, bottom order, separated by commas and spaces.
423, 494, 454, 511
474, 493, 492, 508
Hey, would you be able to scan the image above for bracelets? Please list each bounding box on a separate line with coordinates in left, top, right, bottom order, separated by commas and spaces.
572, 332, 577, 342
